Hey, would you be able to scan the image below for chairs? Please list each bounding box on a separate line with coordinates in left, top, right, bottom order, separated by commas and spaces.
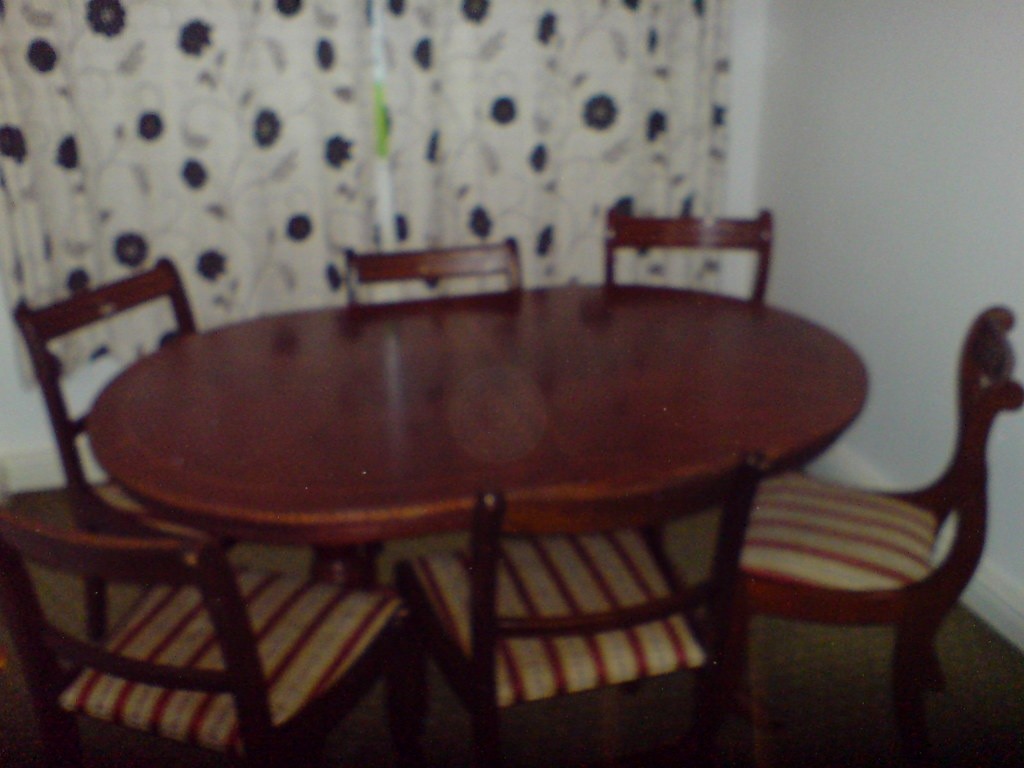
728, 307, 1024, 768
13, 259, 196, 639
605, 208, 772, 302
0, 506, 422, 768
346, 236, 520, 309
392, 461, 761, 768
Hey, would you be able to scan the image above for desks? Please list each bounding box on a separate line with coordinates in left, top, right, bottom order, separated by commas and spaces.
90, 283, 869, 768
